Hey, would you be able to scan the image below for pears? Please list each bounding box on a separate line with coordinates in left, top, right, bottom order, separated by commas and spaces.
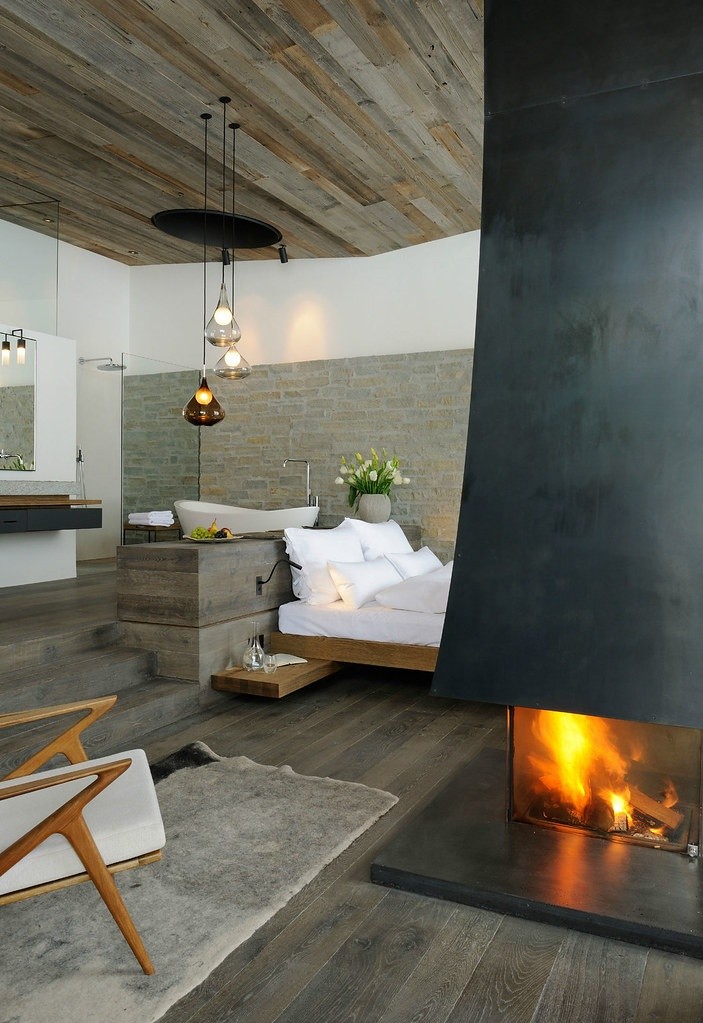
209, 518, 217, 531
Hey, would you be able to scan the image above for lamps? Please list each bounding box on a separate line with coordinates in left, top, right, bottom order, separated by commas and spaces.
2, 334, 9, 368
278, 243, 289, 265
183, 97, 252, 426
12, 329, 26, 365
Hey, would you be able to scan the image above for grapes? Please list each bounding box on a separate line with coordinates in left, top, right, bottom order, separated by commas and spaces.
189, 527, 227, 539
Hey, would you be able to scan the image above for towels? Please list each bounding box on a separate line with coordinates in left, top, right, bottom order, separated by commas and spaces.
128, 510, 174, 527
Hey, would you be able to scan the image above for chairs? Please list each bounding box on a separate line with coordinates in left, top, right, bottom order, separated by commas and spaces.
0, 695, 165, 976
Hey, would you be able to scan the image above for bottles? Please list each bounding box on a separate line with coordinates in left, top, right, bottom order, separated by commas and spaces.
242, 621, 264, 670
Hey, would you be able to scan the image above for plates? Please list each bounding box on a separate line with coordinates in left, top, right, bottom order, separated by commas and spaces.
182, 534, 243, 542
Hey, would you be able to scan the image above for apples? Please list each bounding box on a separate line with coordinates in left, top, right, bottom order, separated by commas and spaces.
221, 528, 231, 535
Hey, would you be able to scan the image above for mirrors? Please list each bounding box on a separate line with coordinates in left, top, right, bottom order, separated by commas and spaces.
0, 332, 36, 471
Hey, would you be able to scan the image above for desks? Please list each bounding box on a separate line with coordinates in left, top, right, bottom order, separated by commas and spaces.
117, 525, 423, 627
123, 523, 182, 545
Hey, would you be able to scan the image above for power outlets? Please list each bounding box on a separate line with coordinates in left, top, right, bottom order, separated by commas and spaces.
256, 575, 263, 596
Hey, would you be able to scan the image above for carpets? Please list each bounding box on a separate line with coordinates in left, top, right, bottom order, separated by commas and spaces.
0, 741, 401, 1023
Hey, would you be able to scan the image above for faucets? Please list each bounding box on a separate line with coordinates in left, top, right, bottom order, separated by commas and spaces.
281, 457, 311, 507
0, 449, 23, 468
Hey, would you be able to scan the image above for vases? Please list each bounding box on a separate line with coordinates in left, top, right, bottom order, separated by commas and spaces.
359, 493, 391, 523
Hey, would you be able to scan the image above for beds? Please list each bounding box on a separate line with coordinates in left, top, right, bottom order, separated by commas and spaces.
269, 600, 445, 673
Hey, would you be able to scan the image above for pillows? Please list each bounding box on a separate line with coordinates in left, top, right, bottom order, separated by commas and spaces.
281, 527, 365, 604
326, 553, 404, 610
384, 546, 444, 580
372, 560, 453, 615
344, 516, 416, 561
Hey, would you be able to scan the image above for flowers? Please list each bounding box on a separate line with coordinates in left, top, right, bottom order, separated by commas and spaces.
335, 445, 410, 515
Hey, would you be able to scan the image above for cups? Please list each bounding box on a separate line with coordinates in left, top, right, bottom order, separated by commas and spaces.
263, 653, 277, 673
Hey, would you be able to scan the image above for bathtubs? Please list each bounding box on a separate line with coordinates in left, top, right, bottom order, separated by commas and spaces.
174, 500, 320, 535
